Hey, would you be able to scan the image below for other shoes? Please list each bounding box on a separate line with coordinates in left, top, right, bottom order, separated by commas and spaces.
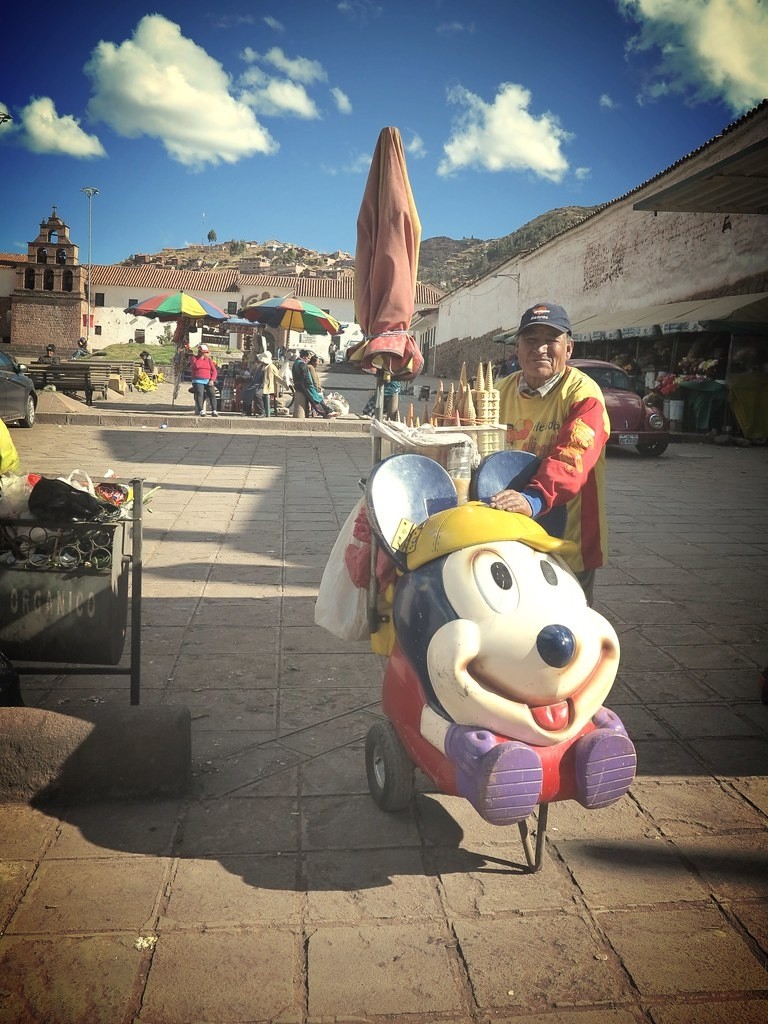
323, 415, 331, 419
256, 413, 265, 418
328, 412, 339, 417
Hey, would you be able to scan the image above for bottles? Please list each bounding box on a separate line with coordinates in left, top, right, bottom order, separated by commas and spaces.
159, 425, 167, 429
446, 442, 471, 506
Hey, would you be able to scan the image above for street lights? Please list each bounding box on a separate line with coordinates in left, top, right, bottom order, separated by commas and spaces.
80, 186, 100, 354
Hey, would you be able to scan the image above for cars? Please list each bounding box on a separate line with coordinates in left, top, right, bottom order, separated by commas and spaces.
565, 358, 671, 457
0, 349, 38, 429
334, 335, 364, 365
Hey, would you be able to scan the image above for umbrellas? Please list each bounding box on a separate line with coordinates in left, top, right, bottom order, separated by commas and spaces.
236, 296, 344, 361
347, 126, 422, 631
123, 290, 231, 407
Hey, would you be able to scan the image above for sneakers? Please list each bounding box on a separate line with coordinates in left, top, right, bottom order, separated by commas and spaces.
199, 410, 206, 416
211, 410, 218, 417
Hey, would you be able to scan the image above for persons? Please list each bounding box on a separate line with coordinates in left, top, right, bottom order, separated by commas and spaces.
191, 345, 218, 417
252, 324, 275, 359
37, 344, 59, 364
489, 303, 611, 609
280, 346, 340, 419
139, 352, 155, 373
328, 343, 338, 365
72, 336, 92, 360
0, 416, 18, 478
240, 351, 291, 418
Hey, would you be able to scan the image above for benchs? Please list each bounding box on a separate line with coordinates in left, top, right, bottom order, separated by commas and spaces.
21, 359, 153, 406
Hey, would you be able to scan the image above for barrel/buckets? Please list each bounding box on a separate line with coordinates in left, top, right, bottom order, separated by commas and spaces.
670, 400, 684, 419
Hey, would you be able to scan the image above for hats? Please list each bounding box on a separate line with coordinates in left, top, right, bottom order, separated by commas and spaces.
514, 302, 574, 337
198, 345, 209, 354
46, 344, 56, 353
80, 337, 85, 342
256, 351, 273, 365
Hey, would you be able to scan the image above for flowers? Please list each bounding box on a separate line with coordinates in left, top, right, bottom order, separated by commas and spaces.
643, 355, 719, 406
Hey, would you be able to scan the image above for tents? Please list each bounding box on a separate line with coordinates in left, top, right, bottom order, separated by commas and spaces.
492, 293, 768, 431
